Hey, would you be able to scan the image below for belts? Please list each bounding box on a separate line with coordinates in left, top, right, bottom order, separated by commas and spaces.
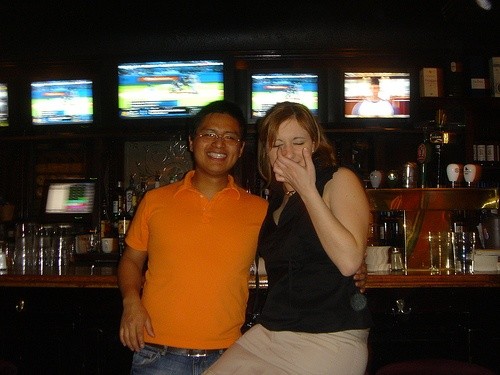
144, 341, 229, 358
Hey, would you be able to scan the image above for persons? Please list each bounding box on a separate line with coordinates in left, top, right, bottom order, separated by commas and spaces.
197, 102, 371, 375
117, 100, 371, 375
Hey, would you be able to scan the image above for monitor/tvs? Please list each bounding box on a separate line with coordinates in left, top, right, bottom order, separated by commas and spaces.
247, 73, 323, 124
117, 59, 228, 121
341, 71, 411, 123
0, 83, 10, 127
31, 80, 95, 127
39, 176, 100, 227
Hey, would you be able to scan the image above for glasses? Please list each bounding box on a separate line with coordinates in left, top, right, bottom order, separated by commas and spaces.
192, 131, 242, 144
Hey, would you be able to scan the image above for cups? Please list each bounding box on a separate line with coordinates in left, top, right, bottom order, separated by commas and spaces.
428, 231, 452, 276
365, 247, 391, 273
451, 232, 476, 276
102, 238, 119, 254
0, 223, 74, 276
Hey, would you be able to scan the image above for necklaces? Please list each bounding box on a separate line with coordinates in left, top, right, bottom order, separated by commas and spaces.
285, 190, 296, 195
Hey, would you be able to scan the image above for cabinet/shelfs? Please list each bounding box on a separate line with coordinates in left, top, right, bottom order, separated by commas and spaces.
0, 131, 500, 272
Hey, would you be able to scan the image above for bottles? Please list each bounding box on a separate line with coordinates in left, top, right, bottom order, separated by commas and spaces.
336, 147, 372, 189
100, 176, 178, 256
367, 211, 404, 248
263, 188, 269, 200
